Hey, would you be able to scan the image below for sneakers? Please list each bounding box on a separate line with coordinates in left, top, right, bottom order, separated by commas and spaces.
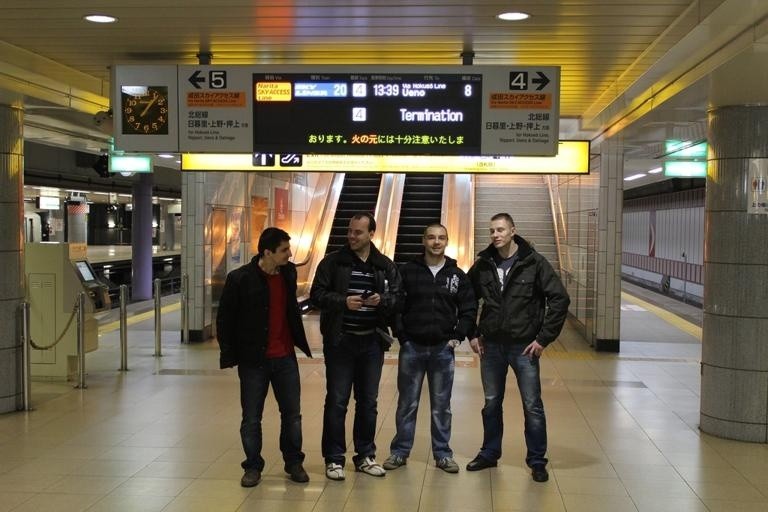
325, 462, 345, 480
355, 456, 386, 477
283, 462, 310, 483
241, 468, 262, 487
383, 453, 407, 470
531, 464, 549, 482
435, 456, 459, 473
465, 454, 497, 472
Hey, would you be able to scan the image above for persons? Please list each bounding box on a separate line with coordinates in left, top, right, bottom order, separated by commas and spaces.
381, 222, 468, 475
461, 211, 571, 484
307, 210, 402, 481
215, 226, 311, 484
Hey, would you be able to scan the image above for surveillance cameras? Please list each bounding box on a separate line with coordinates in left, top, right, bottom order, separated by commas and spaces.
93, 111, 113, 135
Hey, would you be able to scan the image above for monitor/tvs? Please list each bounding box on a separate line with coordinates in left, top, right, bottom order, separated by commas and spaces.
75, 261, 96, 281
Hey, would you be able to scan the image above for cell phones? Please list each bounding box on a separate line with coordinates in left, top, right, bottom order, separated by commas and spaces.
361, 289, 374, 300
